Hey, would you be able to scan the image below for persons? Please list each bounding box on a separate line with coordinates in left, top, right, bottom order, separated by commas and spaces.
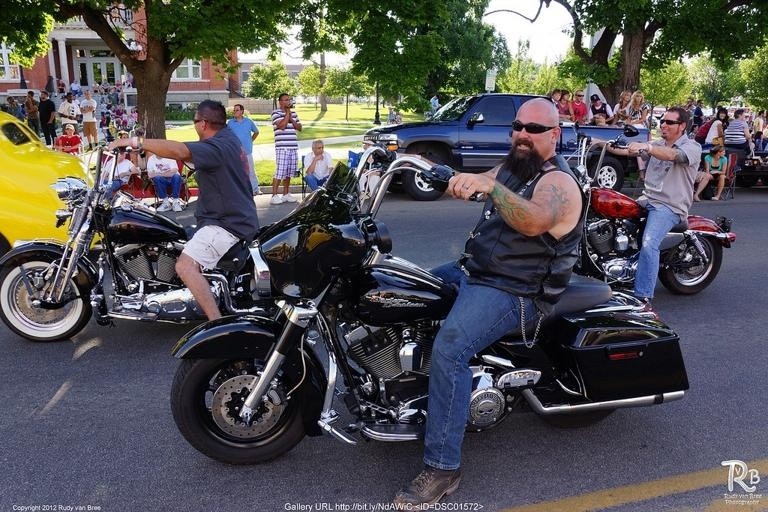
269, 93, 302, 205
358, 139, 383, 198
549, 89, 650, 129
146, 154, 183, 212
99, 78, 139, 138
394, 98, 589, 511
589, 107, 702, 307
6, 79, 98, 154
108, 100, 259, 322
99, 152, 139, 203
228, 104, 263, 197
685, 96, 768, 202
304, 140, 335, 190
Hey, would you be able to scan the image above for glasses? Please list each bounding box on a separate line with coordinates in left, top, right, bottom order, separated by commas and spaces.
660, 119, 683, 125
512, 121, 558, 134
575, 94, 584, 97
194, 119, 210, 123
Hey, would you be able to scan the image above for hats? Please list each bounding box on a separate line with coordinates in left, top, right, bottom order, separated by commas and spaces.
362, 140, 376, 145
65, 124, 75, 131
590, 94, 601, 103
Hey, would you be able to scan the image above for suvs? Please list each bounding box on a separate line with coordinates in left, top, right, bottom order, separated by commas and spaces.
699, 138, 768, 187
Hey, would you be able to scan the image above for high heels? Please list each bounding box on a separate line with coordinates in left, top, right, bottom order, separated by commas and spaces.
693, 195, 702, 203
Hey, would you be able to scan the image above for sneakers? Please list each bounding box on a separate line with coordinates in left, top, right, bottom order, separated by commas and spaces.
156, 201, 183, 213
270, 193, 298, 204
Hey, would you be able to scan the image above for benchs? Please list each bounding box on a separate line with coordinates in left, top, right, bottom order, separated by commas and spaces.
103, 155, 190, 208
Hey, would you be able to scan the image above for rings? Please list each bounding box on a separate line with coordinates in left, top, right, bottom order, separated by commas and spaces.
463, 183, 469, 189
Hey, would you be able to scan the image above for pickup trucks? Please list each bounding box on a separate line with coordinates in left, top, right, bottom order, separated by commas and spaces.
363, 94, 650, 201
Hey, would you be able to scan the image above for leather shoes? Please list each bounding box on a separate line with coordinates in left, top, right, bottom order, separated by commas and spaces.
393, 467, 461, 512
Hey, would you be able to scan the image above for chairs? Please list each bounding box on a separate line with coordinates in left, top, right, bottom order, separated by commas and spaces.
299, 156, 307, 199
716, 153, 737, 200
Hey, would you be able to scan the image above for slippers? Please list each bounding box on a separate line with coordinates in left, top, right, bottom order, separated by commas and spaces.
712, 196, 719, 201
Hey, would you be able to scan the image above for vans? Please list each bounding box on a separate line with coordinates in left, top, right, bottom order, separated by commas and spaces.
0, 111, 106, 269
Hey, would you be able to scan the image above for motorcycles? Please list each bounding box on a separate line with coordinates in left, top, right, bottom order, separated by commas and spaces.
565, 120, 736, 295
170, 144, 690, 463
1, 138, 280, 339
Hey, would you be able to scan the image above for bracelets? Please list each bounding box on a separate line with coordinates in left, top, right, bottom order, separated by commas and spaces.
132, 136, 138, 148
648, 144, 653, 154
139, 137, 144, 148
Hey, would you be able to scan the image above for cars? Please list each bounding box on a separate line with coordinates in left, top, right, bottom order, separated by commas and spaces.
653, 106, 668, 119
